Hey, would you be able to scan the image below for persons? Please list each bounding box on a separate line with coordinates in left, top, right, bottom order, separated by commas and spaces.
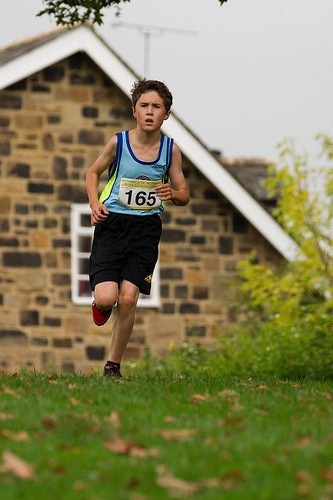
84, 78, 191, 378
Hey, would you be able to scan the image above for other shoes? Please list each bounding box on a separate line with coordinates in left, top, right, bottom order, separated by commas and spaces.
91, 301, 113, 326
